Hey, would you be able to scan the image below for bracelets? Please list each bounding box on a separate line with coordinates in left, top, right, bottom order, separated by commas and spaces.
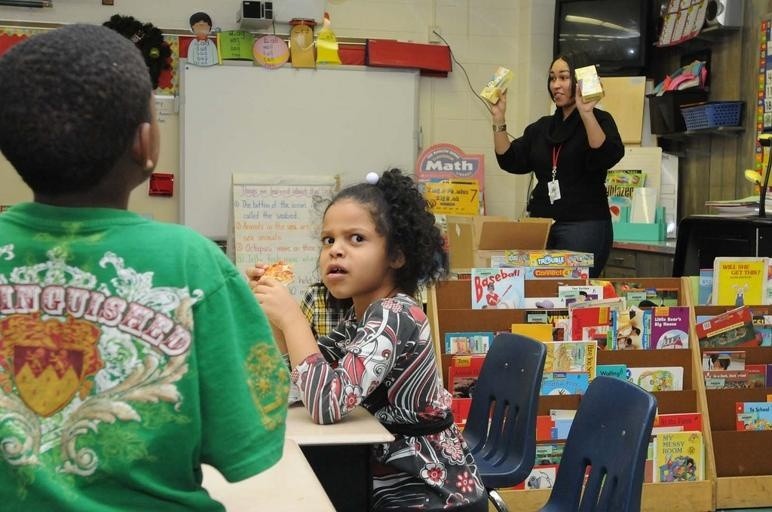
492, 124, 507, 132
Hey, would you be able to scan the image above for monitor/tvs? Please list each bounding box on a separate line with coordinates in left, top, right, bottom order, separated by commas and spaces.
553, 0, 649, 73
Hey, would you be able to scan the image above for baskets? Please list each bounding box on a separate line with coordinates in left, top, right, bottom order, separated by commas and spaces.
679, 102, 744, 131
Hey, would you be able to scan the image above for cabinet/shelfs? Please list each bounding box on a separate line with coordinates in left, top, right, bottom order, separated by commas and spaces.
684, 276, 771, 508
657, 24, 745, 140
427, 277, 715, 511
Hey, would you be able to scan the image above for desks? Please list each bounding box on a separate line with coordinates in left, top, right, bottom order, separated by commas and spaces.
200, 405, 396, 512
601, 237, 677, 277
672, 214, 772, 277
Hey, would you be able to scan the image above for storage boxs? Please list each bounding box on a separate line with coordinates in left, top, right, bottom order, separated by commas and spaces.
446, 215, 556, 273
645, 88, 708, 134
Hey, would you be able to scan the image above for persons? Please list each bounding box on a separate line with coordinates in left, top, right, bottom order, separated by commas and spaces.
1, 21, 293, 512
487, 47, 625, 278
245, 165, 491, 510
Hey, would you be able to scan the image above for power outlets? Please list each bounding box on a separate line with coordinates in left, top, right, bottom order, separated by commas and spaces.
428, 26, 441, 42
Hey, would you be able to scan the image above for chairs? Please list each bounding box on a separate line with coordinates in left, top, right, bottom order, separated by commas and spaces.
536, 375, 658, 512
461, 335, 547, 512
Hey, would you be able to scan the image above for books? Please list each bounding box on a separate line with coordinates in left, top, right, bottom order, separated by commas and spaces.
446, 247, 772, 485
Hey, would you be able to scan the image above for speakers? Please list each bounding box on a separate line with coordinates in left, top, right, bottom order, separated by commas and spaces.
706, 0, 741, 30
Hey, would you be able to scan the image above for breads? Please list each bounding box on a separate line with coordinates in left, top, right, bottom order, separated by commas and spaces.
253, 260, 296, 286
